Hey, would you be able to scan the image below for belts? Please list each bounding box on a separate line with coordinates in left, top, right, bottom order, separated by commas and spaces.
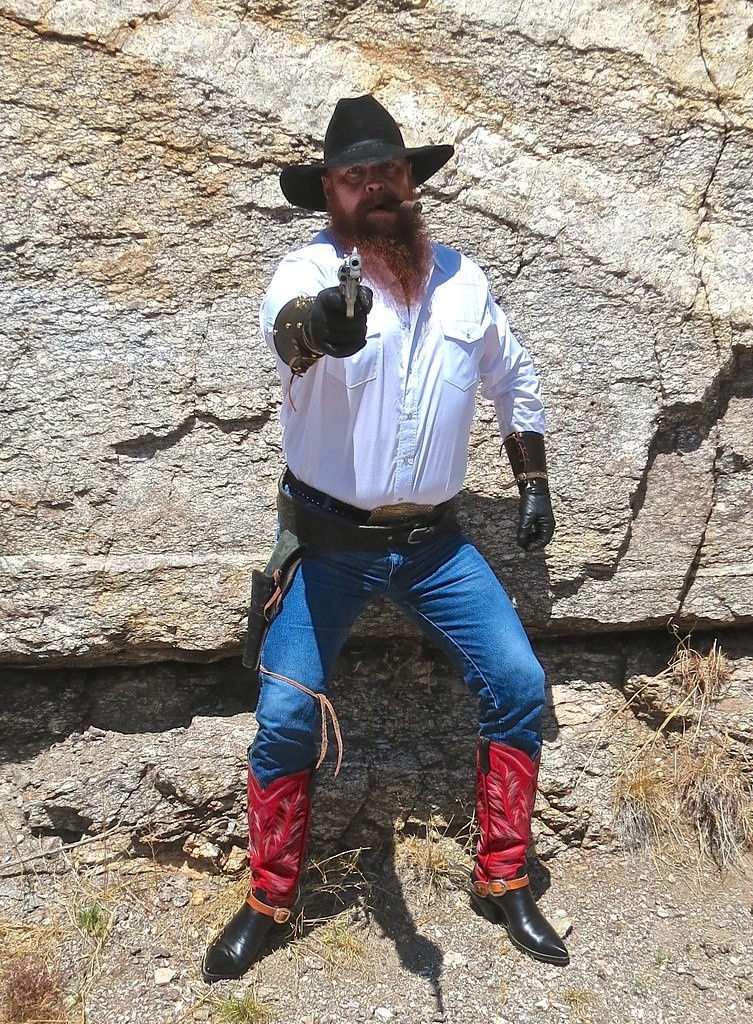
276, 465, 457, 550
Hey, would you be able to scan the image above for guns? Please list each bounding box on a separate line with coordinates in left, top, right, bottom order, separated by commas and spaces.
336, 246, 365, 321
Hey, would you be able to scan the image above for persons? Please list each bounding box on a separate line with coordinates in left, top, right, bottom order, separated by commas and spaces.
205, 90, 571, 979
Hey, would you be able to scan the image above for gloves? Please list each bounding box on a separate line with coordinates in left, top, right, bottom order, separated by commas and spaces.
500, 430, 556, 552
272, 285, 373, 378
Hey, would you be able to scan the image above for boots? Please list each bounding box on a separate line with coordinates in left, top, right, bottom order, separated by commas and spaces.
469, 732, 569, 965
201, 745, 313, 981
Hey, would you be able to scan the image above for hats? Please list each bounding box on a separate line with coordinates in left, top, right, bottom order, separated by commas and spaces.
279, 93, 454, 213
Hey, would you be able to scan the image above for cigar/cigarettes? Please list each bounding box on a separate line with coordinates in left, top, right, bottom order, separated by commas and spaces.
381, 201, 422, 215
